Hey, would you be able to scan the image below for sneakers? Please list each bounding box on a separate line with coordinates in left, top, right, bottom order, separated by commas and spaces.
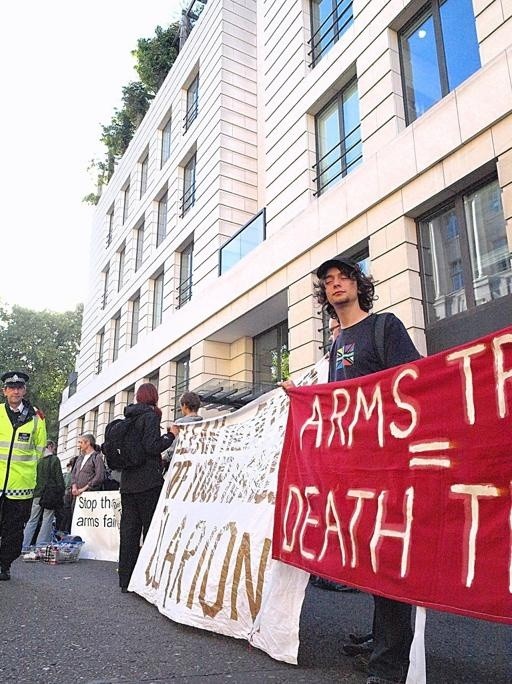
0, 560, 12, 580
339, 630, 406, 683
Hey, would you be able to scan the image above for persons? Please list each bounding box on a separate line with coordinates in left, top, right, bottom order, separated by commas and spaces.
0, 372, 47, 580
63, 383, 204, 593
276, 257, 423, 680
22, 440, 65, 554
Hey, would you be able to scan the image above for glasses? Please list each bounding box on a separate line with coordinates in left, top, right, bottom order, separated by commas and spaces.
327, 324, 340, 336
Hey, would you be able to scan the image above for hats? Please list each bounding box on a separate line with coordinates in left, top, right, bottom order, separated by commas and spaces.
316, 254, 361, 280
1, 371, 30, 388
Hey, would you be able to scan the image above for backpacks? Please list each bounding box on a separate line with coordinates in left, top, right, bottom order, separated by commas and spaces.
100, 412, 143, 472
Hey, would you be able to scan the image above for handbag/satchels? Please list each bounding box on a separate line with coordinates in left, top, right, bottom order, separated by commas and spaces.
38, 455, 65, 510
